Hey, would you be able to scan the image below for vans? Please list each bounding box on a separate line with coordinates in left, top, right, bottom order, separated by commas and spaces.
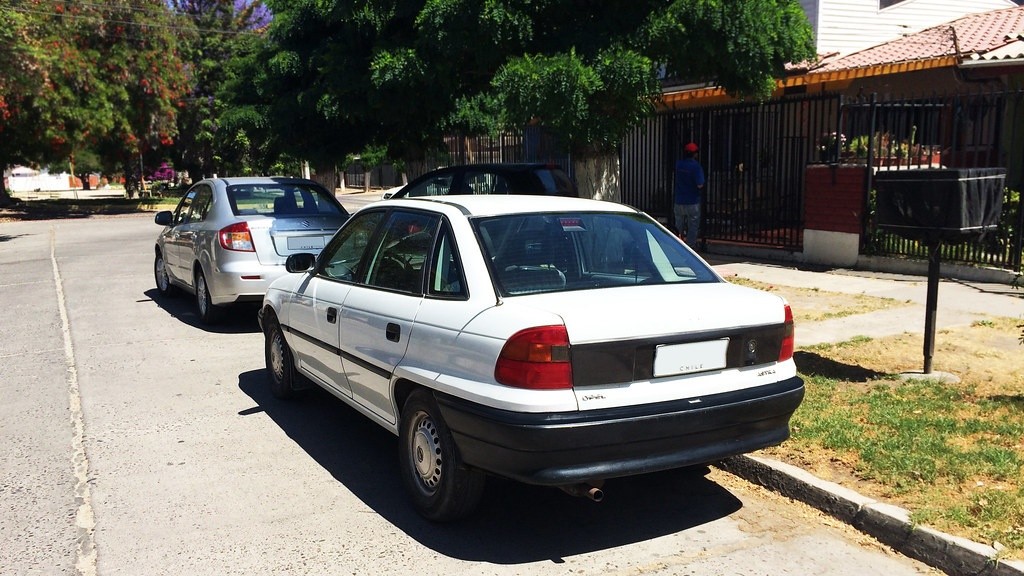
384, 162, 580, 198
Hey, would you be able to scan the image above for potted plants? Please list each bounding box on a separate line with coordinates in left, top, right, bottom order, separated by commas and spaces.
815, 132, 941, 166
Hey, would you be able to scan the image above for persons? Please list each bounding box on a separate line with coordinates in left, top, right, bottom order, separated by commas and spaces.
674, 142, 703, 249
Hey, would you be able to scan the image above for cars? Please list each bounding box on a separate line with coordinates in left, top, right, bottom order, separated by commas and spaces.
155, 177, 353, 325
259, 194, 806, 523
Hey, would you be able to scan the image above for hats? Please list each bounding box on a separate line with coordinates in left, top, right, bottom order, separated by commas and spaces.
685, 143, 698, 151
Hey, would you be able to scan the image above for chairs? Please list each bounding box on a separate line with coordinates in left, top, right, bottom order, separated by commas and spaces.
498, 229, 567, 291
274, 197, 298, 213
